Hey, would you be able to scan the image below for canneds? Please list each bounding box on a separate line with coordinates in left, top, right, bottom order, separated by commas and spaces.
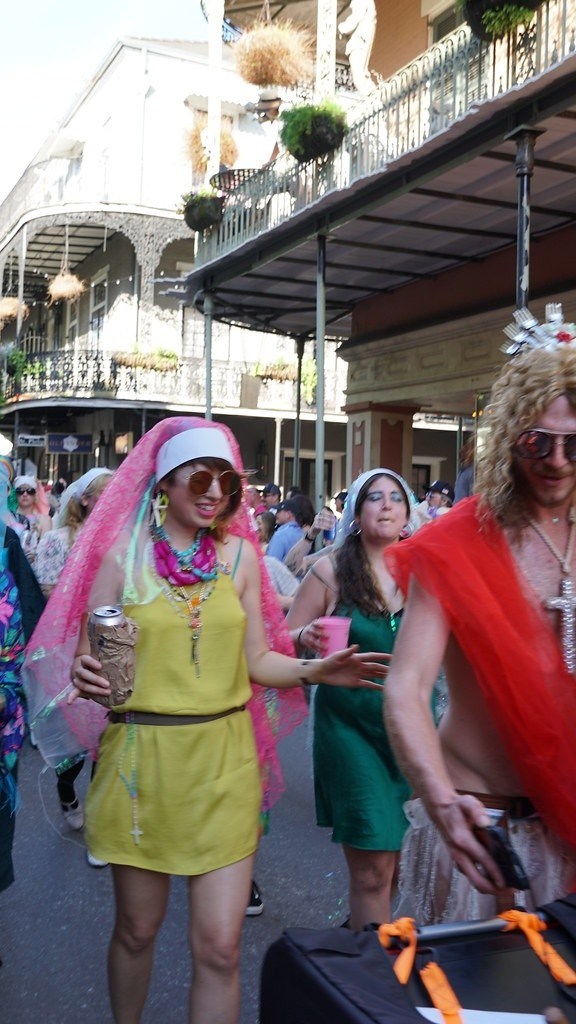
324, 515, 337, 540
89, 606, 129, 649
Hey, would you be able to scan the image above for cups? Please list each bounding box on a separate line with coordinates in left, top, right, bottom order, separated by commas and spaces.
319, 616, 351, 658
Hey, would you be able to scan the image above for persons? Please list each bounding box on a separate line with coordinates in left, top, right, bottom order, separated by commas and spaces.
339, 0, 378, 99
240, 482, 352, 918
275, 468, 446, 935
255, 91, 315, 229
0, 456, 122, 888
379, 342, 576, 931
412, 435, 478, 522
18, 415, 395, 1024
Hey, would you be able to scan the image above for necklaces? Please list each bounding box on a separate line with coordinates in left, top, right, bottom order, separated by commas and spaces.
519, 505, 576, 672
146, 519, 217, 680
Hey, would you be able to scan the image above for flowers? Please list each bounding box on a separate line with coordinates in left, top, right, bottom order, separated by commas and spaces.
177, 191, 224, 217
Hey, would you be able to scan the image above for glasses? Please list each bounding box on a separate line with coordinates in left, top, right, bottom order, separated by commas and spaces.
426, 492, 442, 498
16, 487, 36, 495
176, 470, 241, 497
515, 429, 576, 459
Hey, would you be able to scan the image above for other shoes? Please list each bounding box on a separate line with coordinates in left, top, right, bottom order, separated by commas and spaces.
87, 850, 109, 867
59, 785, 84, 830
244, 881, 265, 915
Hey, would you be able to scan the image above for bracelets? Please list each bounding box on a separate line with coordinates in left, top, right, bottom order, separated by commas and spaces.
305, 533, 316, 541
297, 659, 318, 686
297, 624, 305, 647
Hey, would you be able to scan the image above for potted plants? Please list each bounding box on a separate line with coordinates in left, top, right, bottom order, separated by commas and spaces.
452, 0, 540, 42
280, 104, 348, 162
6, 349, 26, 377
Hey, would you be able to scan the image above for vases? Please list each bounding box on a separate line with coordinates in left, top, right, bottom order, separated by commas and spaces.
184, 197, 222, 232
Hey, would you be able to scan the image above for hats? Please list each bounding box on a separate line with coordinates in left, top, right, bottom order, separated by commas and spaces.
257, 484, 281, 494
421, 480, 455, 502
271, 500, 299, 513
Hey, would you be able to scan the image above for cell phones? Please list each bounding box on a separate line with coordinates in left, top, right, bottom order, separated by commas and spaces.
457, 825, 531, 892
